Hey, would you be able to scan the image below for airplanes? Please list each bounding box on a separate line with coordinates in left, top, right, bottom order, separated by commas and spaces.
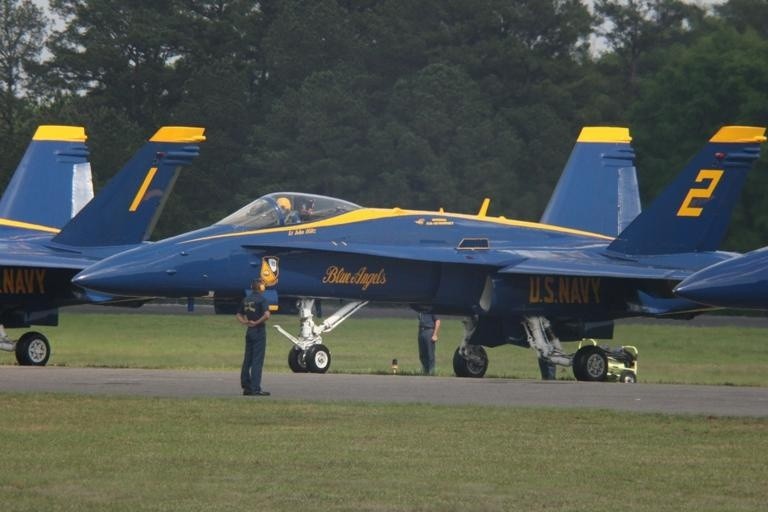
71, 125, 768, 381
0, 124, 206, 366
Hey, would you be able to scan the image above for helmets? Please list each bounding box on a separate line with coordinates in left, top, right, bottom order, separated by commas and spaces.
276, 198, 291, 211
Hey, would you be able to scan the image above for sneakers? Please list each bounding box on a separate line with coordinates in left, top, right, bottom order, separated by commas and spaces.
243, 389, 270, 396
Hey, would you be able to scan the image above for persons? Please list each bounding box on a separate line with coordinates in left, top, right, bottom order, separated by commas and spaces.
236, 279, 271, 396
417, 312, 440, 374
276, 197, 301, 225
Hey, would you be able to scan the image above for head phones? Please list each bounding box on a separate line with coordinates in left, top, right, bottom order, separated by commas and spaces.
250, 280, 266, 292
